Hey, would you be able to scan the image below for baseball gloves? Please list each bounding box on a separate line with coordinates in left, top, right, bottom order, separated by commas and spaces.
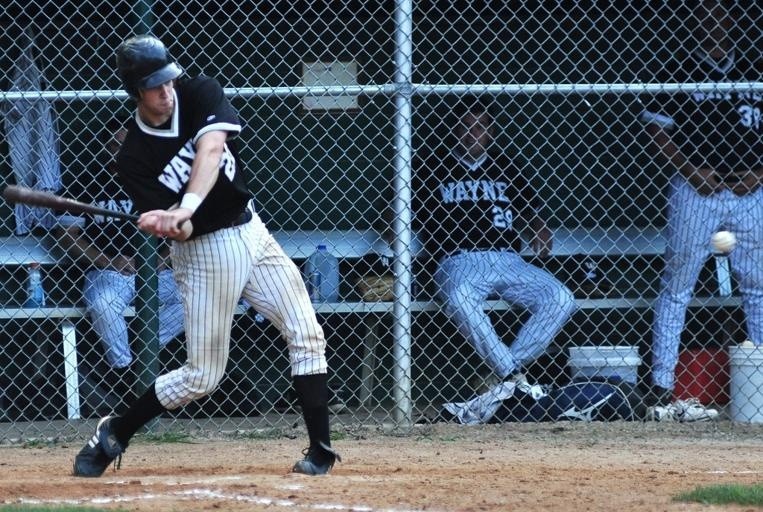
355, 275, 397, 302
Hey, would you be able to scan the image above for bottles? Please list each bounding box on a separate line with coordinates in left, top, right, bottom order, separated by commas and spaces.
304, 243, 340, 302
26, 267, 45, 307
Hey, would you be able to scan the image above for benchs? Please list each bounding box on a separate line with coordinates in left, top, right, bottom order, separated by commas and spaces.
0, 224, 744, 420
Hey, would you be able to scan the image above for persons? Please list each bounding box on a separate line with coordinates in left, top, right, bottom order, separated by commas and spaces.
411, 105, 576, 402
50, 115, 187, 413
72, 34, 342, 479
645, 0, 762, 425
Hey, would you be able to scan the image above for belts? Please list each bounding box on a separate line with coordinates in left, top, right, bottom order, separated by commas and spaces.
190, 207, 251, 237
444, 248, 517, 259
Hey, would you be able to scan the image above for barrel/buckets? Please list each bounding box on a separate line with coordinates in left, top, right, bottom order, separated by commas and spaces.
728, 345, 763, 425
568, 346, 641, 388
304, 244, 339, 302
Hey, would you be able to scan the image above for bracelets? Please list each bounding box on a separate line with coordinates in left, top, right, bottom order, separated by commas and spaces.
178, 192, 203, 215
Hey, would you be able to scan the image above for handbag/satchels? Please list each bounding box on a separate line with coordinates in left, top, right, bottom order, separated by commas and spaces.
525, 376, 648, 422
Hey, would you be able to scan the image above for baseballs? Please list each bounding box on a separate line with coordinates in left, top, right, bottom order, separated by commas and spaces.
711, 230, 735, 255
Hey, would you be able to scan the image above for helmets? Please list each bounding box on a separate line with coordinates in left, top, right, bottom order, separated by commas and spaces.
114, 34, 180, 103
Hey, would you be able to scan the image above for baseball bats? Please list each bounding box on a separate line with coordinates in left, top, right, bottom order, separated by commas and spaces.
3, 184, 182, 231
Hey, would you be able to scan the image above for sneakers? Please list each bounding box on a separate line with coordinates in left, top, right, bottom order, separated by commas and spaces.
646, 385, 672, 405
676, 397, 717, 421
646, 402, 677, 421
328, 384, 347, 413
292, 438, 342, 475
72, 414, 124, 476
473, 375, 493, 394
510, 372, 531, 393
273, 391, 301, 413
